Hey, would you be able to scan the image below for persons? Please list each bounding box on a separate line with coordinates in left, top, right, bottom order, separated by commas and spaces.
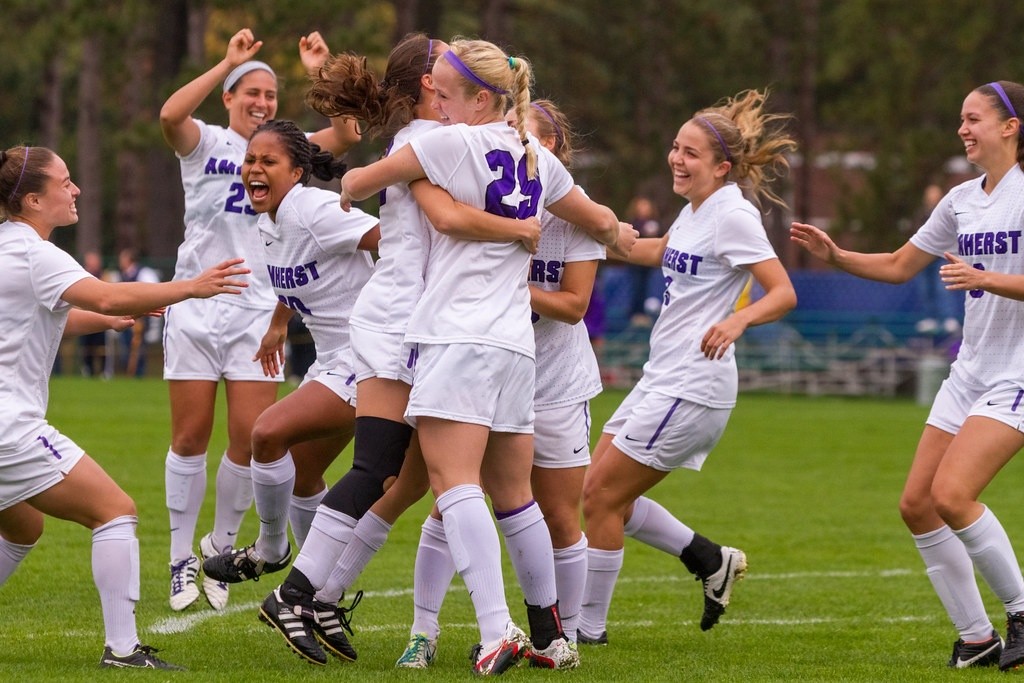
826, 165, 899, 253
907, 157, 985, 331
161, 29, 660, 677
578, 88, 797, 649
77, 247, 160, 377
790, 81, 1024, 673
0, 146, 251, 672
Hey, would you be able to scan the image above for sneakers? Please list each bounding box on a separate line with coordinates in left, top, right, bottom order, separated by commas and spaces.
311, 590, 358, 664
948, 629, 1005, 670
395, 632, 438, 670
257, 585, 327, 666
202, 539, 294, 583
197, 532, 230, 611
98, 644, 187, 672
701, 545, 747, 632
576, 628, 608, 646
469, 619, 531, 677
998, 611, 1024, 670
168, 551, 201, 612
528, 637, 580, 669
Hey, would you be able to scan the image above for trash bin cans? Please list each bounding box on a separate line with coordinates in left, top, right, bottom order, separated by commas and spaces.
912, 355, 950, 408
